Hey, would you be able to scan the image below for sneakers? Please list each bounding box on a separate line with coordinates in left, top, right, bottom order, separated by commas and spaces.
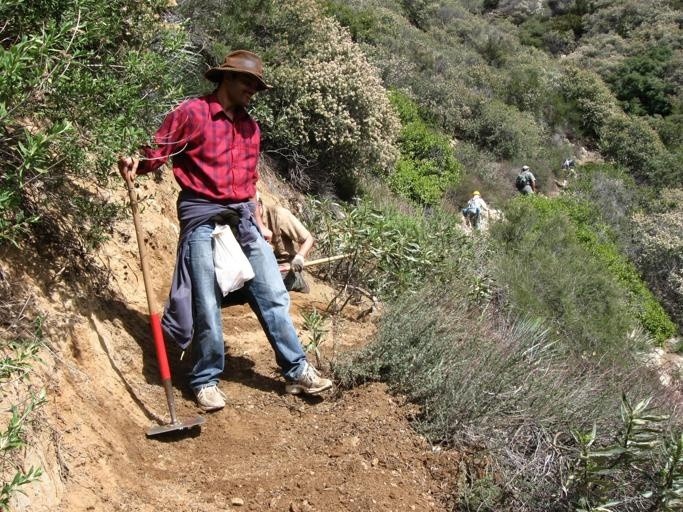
285, 358, 333, 395
192, 371, 226, 411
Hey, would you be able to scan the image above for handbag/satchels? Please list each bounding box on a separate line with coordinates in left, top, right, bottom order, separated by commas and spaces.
209, 222, 256, 298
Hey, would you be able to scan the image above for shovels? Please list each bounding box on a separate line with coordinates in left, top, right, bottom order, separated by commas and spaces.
121, 168, 205, 437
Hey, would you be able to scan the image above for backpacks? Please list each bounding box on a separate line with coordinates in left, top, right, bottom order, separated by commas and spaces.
515, 172, 530, 190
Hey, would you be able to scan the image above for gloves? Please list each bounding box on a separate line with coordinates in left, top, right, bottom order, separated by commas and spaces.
290, 252, 304, 273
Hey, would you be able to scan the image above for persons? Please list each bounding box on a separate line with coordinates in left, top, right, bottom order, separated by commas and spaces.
515, 165, 536, 195
464, 190, 490, 230
115, 49, 333, 412
255, 189, 315, 295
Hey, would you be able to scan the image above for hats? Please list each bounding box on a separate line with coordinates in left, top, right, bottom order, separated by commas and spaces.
523, 165, 530, 169
472, 190, 481, 196
203, 50, 274, 89
